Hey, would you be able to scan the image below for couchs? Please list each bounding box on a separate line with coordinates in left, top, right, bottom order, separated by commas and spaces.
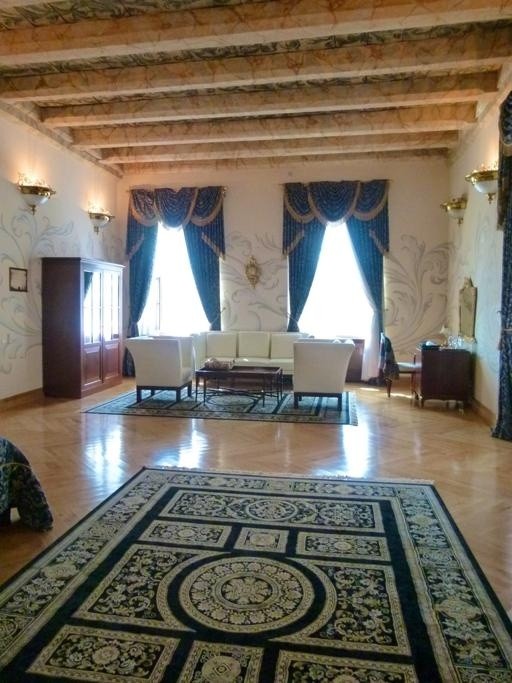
123, 335, 195, 403
289, 337, 356, 412
198, 329, 316, 374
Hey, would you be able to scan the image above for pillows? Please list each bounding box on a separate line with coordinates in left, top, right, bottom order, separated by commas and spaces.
206, 333, 236, 359
237, 330, 270, 359
271, 334, 297, 360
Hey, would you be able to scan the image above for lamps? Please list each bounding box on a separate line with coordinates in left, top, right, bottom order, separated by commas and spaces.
18, 169, 56, 216
88, 204, 115, 234
439, 190, 467, 227
465, 161, 499, 206
240, 254, 263, 289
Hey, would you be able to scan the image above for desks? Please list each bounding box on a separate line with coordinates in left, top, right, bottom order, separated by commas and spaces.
413, 341, 474, 411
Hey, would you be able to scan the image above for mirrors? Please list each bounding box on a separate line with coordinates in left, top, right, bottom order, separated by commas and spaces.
456, 276, 477, 340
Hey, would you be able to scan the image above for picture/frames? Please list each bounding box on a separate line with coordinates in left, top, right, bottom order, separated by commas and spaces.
8, 266, 29, 292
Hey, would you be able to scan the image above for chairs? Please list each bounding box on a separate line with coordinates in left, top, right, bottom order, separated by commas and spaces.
377, 332, 416, 399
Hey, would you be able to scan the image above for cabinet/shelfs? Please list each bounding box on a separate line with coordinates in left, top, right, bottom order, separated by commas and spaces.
41, 256, 126, 402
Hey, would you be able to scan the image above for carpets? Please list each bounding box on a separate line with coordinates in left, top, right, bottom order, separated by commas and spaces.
78, 388, 360, 427
1, 465, 512, 682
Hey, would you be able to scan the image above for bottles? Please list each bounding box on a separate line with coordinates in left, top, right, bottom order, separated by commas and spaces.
448, 336, 463, 349
